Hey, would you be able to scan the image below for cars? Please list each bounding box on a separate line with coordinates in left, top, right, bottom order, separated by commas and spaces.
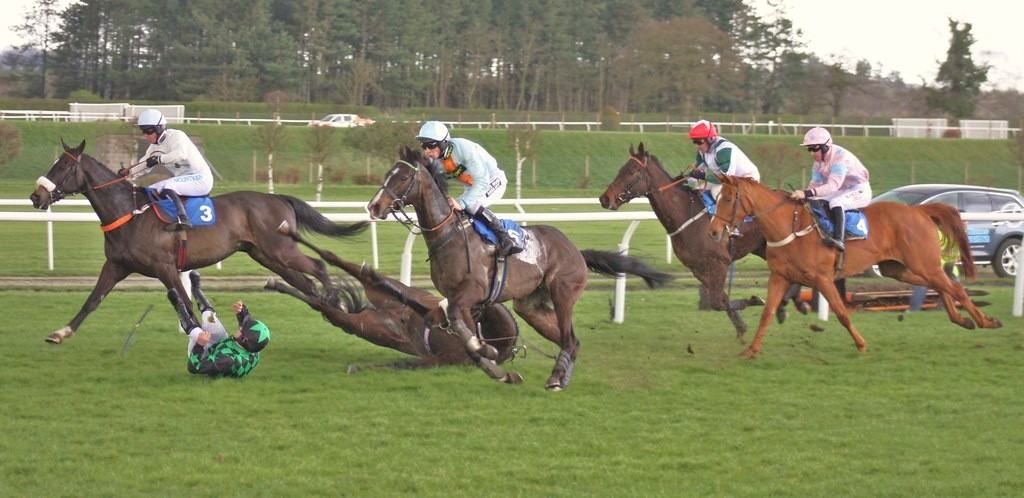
308, 113, 376, 129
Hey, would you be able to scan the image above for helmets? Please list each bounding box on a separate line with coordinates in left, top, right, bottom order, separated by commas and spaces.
416, 121, 450, 141
239, 315, 270, 352
133, 109, 167, 125
800, 128, 832, 146
689, 120, 718, 138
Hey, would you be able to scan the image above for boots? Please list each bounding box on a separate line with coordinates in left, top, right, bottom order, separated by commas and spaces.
474, 205, 524, 255
167, 288, 201, 334
826, 206, 846, 252
190, 271, 214, 313
161, 189, 192, 231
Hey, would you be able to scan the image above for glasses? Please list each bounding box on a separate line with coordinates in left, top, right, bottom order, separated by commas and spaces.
421, 141, 438, 149
693, 139, 707, 144
807, 145, 821, 152
143, 128, 155, 135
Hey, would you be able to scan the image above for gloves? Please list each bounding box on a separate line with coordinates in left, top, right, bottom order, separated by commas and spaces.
147, 155, 161, 167
691, 169, 705, 180
119, 168, 129, 176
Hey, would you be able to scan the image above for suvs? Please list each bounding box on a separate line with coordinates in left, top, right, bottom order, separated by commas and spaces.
866, 183, 1024, 278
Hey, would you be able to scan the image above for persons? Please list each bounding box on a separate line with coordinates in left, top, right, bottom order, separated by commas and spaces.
414, 121, 523, 257
118, 109, 214, 231
789, 127, 872, 252
167, 271, 270, 378
906, 222, 961, 313
674, 120, 760, 204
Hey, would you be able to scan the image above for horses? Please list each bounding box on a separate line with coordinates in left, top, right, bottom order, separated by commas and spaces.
367, 145, 678, 392
599, 141, 811, 340
29, 135, 369, 344
264, 221, 518, 365
707, 169, 1004, 359
